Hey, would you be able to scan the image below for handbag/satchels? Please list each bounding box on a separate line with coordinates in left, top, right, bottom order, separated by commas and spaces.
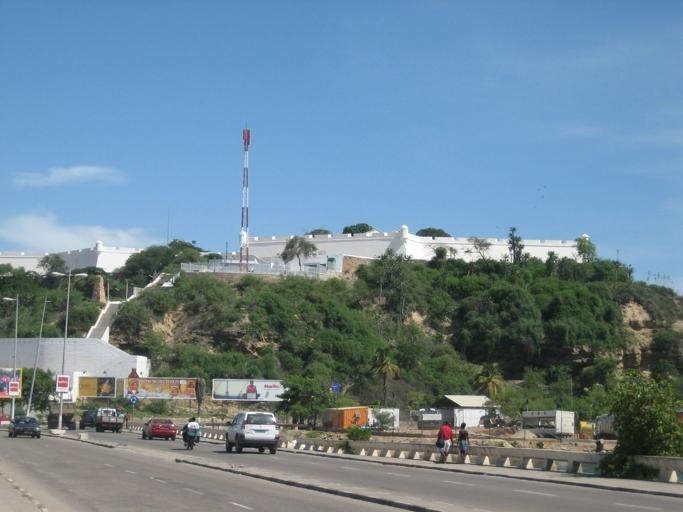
436, 433, 445, 448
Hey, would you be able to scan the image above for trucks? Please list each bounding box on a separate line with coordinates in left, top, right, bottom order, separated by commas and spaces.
415, 408, 442, 431
47, 402, 123, 434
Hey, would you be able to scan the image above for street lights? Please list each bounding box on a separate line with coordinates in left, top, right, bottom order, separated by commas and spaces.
2, 267, 91, 429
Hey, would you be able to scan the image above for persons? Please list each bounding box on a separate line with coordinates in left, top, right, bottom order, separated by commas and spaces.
123, 411, 130, 429
456, 422, 471, 464
186, 416, 199, 443
435, 419, 454, 466
180, 418, 200, 447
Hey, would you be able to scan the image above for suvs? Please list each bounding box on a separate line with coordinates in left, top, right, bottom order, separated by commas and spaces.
222, 411, 277, 456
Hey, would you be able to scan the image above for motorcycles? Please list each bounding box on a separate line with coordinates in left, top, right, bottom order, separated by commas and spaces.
180, 426, 199, 451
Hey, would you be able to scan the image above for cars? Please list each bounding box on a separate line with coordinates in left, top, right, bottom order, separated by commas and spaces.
7, 416, 40, 437
140, 417, 176, 441
